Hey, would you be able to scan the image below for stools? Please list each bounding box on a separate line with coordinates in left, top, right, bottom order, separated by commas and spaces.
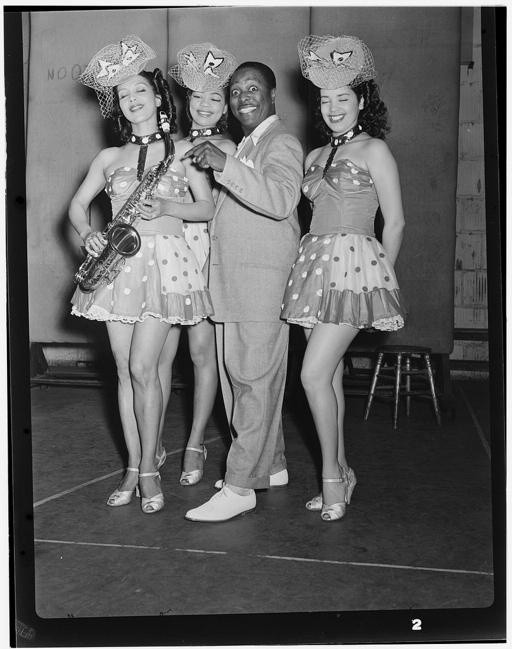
364, 346, 443, 431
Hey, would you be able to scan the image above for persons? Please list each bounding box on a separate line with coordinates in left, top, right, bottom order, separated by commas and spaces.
153, 86, 241, 487
279, 81, 408, 521
68, 70, 216, 514
178, 61, 304, 522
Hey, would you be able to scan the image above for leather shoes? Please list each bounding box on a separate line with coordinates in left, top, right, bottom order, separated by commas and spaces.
213, 467, 289, 489
184, 482, 257, 524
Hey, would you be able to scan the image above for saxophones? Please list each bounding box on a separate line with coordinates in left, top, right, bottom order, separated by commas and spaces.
73, 110, 175, 293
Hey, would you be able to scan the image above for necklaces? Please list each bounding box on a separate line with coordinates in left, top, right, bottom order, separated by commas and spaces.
330, 123, 362, 147
189, 127, 221, 137
129, 133, 165, 144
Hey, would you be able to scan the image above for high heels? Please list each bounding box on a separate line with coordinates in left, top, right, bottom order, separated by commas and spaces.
155, 446, 167, 470
106, 467, 141, 507
320, 461, 350, 521
179, 445, 208, 486
305, 466, 357, 511
138, 470, 165, 514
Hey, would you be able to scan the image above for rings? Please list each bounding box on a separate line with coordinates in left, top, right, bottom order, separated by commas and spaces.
198, 155, 202, 160
86, 237, 92, 242
